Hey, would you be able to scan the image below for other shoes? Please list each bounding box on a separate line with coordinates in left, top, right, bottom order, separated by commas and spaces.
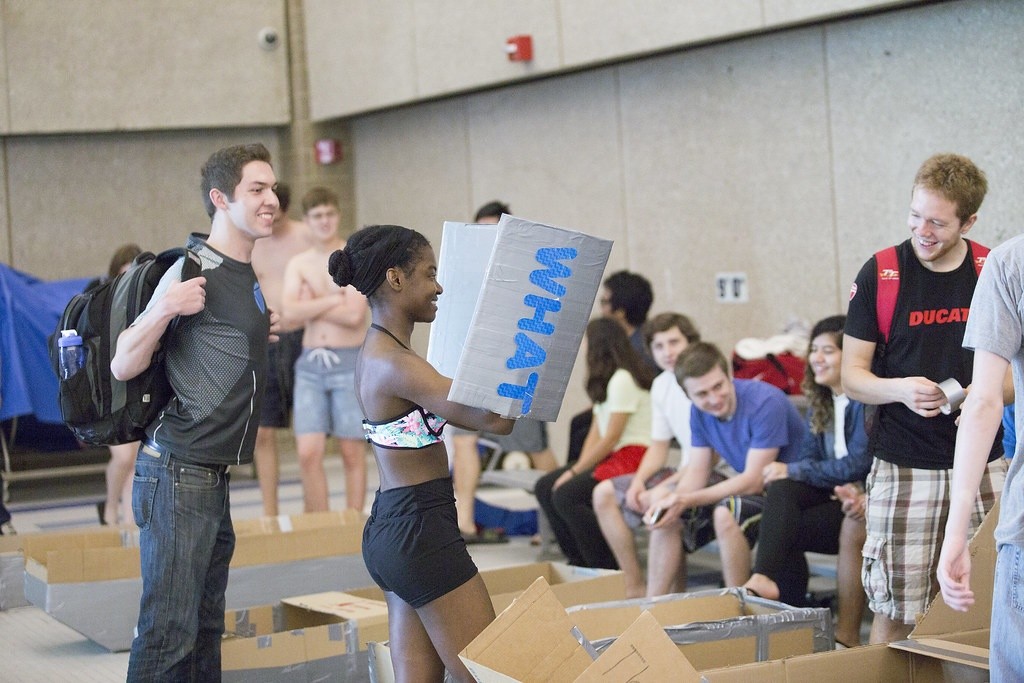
97, 501, 105, 523
458, 529, 508, 544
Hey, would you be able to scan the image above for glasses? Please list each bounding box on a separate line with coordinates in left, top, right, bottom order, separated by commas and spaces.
600, 297, 617, 306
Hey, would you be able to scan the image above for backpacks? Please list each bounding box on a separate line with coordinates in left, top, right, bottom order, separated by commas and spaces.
47, 249, 202, 447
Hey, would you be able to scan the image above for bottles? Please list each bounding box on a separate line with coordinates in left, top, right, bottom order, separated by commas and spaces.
57, 329, 85, 382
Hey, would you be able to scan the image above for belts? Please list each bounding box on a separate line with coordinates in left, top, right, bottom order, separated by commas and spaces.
141, 434, 163, 450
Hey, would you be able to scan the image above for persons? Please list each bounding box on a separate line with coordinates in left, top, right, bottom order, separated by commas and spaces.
539, 271, 662, 563
327, 224, 517, 683
643, 341, 811, 601
278, 188, 376, 518
108, 142, 281, 683
554, 317, 662, 572
443, 202, 562, 545
735, 315, 877, 651
247, 183, 315, 515
840, 153, 1009, 649
592, 314, 737, 599
833, 233, 1023, 683
96, 242, 161, 525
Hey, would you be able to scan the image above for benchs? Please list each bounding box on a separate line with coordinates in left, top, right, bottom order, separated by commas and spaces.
486, 470, 837, 578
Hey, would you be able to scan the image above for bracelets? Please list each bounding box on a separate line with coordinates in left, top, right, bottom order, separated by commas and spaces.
568, 467, 577, 479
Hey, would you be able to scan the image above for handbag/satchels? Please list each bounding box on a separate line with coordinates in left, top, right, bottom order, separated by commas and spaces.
732, 352, 809, 394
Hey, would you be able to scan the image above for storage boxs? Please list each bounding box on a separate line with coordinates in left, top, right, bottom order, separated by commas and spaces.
0, 493, 1001, 683
